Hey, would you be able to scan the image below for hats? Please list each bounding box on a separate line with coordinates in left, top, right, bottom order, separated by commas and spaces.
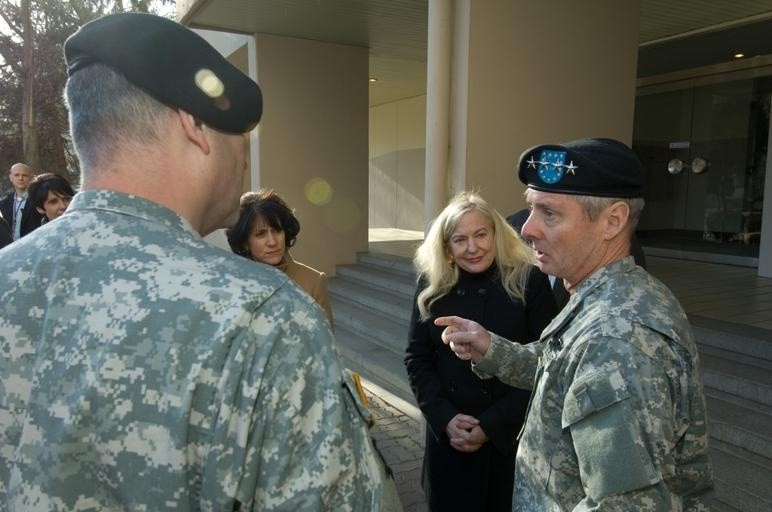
518, 136, 653, 199
64, 11, 262, 133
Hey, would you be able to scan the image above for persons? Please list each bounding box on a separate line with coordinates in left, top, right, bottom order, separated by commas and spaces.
225, 187, 335, 335
0, 162, 48, 249
404, 188, 557, 512
27, 172, 75, 226
1, 10, 404, 512
432, 137, 716, 512
